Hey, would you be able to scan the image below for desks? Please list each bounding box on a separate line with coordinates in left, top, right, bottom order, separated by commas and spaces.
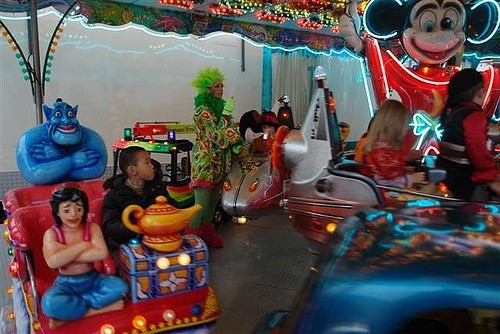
110, 139, 194, 186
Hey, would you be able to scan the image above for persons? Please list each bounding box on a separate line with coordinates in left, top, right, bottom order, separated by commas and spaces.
42, 189, 129, 320
354, 99, 427, 189
249, 110, 279, 158
16, 99, 107, 184
190, 66, 247, 251
100, 146, 187, 255
434, 67, 500, 201
